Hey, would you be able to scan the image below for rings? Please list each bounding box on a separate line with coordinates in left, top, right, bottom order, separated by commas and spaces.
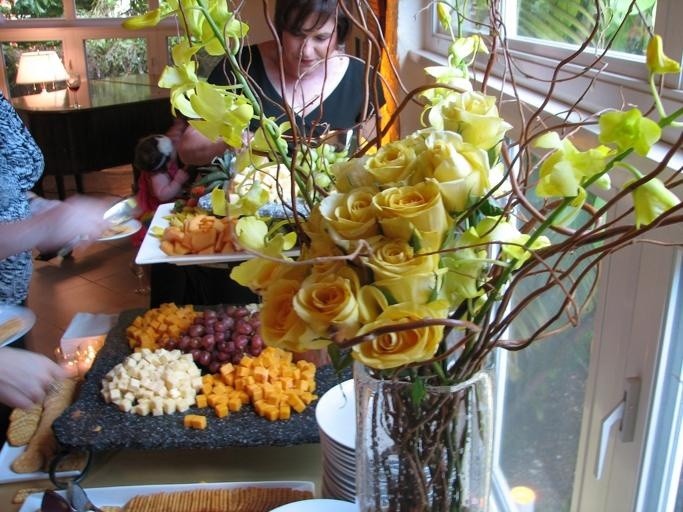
45, 381, 64, 396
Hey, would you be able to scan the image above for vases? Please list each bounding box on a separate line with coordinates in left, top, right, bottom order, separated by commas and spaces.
351, 344, 510, 511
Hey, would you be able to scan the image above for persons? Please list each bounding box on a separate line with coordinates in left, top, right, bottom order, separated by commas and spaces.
174, 1, 395, 307
0, 91, 126, 452
130, 117, 190, 249
0, 345, 68, 414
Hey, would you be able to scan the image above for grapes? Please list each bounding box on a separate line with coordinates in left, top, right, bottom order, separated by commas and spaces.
167, 305, 264, 374
291, 143, 344, 188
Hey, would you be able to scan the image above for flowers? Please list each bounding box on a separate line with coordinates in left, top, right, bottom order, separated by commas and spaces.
122, 0, 683, 511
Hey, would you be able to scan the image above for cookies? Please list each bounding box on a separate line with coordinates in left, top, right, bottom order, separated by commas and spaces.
13, 487, 53, 504
159, 214, 243, 255
97, 486, 313, 512
6, 374, 86, 473
0, 318, 24, 345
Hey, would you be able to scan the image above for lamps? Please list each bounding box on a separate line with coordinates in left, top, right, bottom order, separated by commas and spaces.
14, 49, 71, 95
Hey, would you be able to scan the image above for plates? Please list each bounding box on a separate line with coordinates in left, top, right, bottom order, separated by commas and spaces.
19, 480, 316, 512
313, 378, 355, 505
0, 443, 92, 483
0, 304, 37, 349
98, 219, 143, 241
133, 202, 303, 265
